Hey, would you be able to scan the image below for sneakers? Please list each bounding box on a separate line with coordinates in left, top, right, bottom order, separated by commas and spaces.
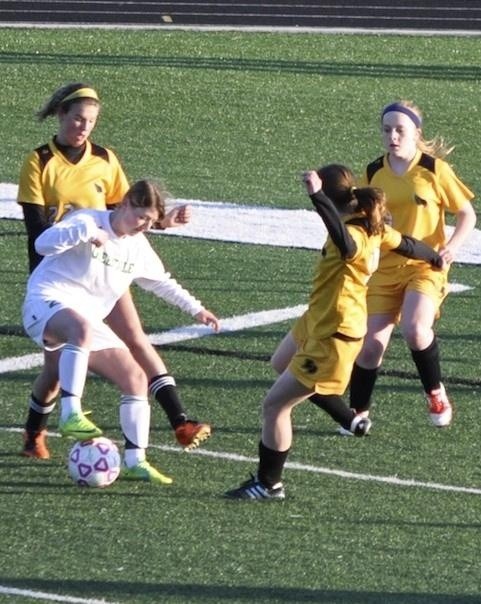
120, 462, 171, 486
57, 414, 101, 438
176, 422, 210, 451
334, 410, 371, 437
22, 435, 49, 459
219, 472, 285, 502
427, 383, 454, 427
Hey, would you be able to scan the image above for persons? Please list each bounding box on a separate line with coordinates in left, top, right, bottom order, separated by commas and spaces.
340, 98, 478, 437
219, 162, 447, 503
21, 177, 222, 485
17, 83, 215, 458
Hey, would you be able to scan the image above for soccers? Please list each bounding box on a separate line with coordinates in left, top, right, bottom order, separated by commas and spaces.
69, 436, 121, 488
